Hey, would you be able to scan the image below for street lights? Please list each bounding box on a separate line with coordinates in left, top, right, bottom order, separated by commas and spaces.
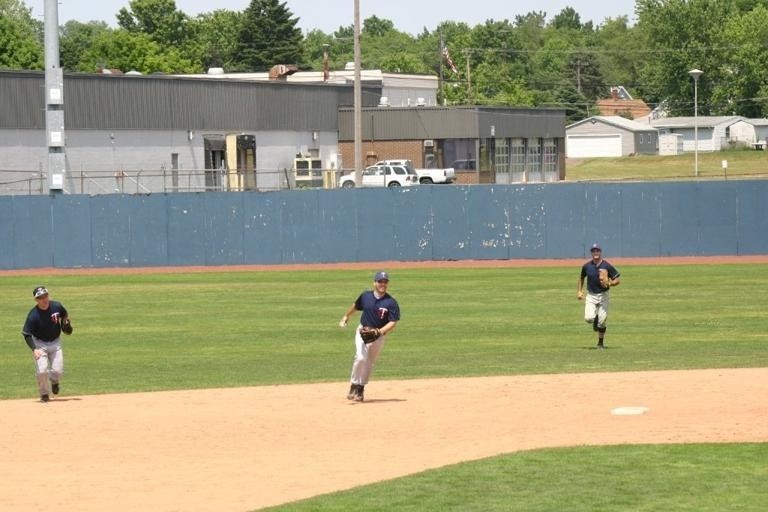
688, 69, 704, 176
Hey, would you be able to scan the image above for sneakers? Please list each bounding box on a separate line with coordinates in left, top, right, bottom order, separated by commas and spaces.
52, 383, 59, 394
347, 384, 364, 401
41, 394, 49, 401
597, 342, 603, 350
593, 315, 599, 331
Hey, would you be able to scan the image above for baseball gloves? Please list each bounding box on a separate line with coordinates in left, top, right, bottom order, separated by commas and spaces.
599, 268, 611, 288
61, 318, 73, 335
360, 327, 384, 344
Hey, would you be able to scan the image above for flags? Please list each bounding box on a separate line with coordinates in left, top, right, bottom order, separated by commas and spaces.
442, 45, 460, 74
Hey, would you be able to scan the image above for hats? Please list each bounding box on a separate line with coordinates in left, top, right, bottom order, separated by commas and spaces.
33, 286, 48, 298
374, 271, 389, 281
591, 244, 601, 252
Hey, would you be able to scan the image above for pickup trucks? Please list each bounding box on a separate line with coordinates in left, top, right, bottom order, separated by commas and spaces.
375, 159, 457, 184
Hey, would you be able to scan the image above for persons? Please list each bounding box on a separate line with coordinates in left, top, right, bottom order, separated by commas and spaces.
21, 286, 73, 403
338, 272, 401, 403
576, 244, 621, 351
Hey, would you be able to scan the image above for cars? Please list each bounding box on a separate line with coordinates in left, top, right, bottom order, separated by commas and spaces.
448, 159, 476, 170
339, 164, 420, 188
